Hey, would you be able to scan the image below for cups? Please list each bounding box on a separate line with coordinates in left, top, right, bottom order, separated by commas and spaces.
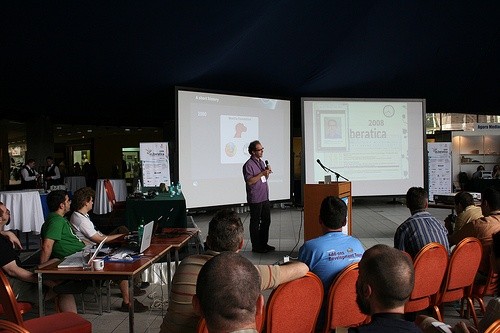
92, 259, 104, 270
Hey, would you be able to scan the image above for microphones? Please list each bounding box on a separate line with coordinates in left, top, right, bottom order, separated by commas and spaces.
316, 159, 326, 171
265, 160, 271, 171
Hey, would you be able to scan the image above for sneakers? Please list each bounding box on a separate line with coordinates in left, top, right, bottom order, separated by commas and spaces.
252, 244, 275, 253
134, 285, 146, 296
139, 282, 149, 289
121, 298, 148, 313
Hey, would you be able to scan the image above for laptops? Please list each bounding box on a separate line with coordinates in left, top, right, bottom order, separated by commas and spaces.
57, 237, 108, 268
128, 216, 163, 242
117, 220, 154, 253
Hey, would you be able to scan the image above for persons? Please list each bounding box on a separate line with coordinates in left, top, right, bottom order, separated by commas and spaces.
21, 158, 40, 190
0, 201, 77, 314
326, 120, 340, 139
192, 252, 264, 333
492, 165, 500, 179
70, 187, 150, 296
160, 211, 310, 333
298, 195, 365, 332
414, 230, 500, 333
131, 155, 141, 178
59, 156, 67, 176
444, 191, 485, 238
348, 244, 424, 333
473, 165, 485, 178
242, 140, 276, 254
81, 153, 89, 169
394, 187, 450, 261
448, 187, 500, 275
39, 190, 148, 313
45, 155, 61, 184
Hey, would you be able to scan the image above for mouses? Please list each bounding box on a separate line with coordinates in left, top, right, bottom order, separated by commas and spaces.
128, 241, 138, 245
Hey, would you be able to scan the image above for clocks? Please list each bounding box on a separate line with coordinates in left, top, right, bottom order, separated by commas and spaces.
383, 105, 395, 118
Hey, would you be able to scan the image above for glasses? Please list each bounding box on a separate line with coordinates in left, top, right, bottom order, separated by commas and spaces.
255, 148, 264, 151
63, 199, 71, 205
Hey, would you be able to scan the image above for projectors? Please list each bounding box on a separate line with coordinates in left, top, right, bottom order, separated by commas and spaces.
433, 191, 481, 205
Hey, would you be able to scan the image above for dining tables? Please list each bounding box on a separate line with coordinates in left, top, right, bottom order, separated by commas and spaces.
40, 191, 73, 218
0, 190, 45, 250
63, 176, 86, 195
93, 179, 126, 215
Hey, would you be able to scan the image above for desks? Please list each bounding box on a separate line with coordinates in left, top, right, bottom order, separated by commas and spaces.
127, 187, 187, 230
108, 228, 200, 273
34, 244, 173, 333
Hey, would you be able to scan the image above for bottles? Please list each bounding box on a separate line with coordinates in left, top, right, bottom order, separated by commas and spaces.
136, 180, 141, 191
169, 182, 174, 197
137, 216, 146, 251
177, 181, 181, 195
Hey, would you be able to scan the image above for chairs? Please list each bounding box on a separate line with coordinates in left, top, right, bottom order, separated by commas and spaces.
196, 236, 500, 333
0, 269, 112, 333
103, 179, 126, 210
